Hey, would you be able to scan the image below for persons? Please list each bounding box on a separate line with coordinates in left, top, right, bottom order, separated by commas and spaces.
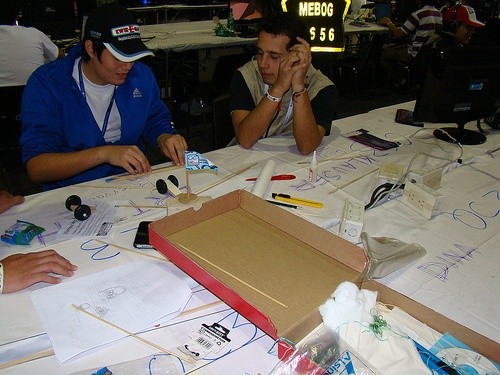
225, 12, 337, 155
0, 0, 66, 119
21, 0, 189, 182
0, 190, 78, 295
411, 1, 500, 122
379, 0, 442, 83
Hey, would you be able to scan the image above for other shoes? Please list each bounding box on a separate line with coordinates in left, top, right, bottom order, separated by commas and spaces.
394, 78, 406, 87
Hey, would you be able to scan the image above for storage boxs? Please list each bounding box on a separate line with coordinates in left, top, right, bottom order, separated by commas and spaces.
148, 189, 499, 375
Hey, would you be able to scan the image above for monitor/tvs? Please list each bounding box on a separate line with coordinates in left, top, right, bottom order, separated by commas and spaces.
412, 65, 500, 145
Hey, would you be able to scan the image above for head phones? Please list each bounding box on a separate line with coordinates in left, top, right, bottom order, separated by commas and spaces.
446, 3, 462, 32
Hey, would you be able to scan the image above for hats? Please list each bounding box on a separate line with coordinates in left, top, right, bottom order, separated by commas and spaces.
85, 2, 155, 62
444, 5, 485, 27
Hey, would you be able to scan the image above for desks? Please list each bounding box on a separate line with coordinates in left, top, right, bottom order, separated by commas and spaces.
344, 19, 390, 70
0, 97, 500, 375
126, 5, 259, 103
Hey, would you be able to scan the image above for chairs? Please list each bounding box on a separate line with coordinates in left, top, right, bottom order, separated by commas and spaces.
418, 40, 447, 76
395, 44, 431, 94
211, 97, 235, 149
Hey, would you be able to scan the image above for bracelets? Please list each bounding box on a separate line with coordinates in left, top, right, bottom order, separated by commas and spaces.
0, 262, 4, 292
291, 87, 307, 103
386, 21, 392, 26
265, 89, 282, 102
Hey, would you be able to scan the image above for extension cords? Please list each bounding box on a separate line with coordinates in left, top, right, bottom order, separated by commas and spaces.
402, 183, 445, 219
338, 198, 365, 244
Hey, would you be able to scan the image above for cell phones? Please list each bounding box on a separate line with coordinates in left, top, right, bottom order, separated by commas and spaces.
133, 221, 154, 248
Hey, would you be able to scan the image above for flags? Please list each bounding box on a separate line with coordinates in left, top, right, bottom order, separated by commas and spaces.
185, 151, 218, 174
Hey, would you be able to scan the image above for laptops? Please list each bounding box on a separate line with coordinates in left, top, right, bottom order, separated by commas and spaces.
375, 2, 403, 27
